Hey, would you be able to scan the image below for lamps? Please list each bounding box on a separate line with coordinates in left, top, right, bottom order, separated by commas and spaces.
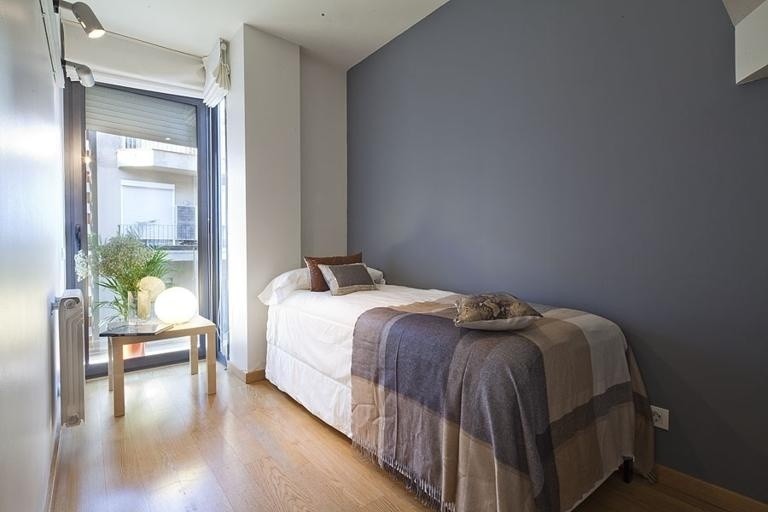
153, 287, 197, 323
39, 0, 106, 89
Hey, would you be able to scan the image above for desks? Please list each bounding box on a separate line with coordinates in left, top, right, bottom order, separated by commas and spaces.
108, 314, 216, 418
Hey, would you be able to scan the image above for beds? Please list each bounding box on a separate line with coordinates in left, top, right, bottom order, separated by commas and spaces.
257, 268, 655, 511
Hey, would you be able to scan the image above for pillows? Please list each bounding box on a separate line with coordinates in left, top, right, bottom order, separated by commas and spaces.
453, 292, 544, 331
304, 252, 361, 292
318, 262, 379, 296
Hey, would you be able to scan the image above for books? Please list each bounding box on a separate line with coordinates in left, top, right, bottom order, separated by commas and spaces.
99, 324, 173, 335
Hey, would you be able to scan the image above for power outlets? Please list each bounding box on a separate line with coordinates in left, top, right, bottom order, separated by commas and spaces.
650, 405, 669, 431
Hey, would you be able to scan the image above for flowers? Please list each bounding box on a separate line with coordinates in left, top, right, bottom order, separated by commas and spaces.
91, 236, 177, 327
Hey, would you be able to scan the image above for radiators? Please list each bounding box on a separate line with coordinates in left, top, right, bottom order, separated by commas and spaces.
51, 289, 85, 428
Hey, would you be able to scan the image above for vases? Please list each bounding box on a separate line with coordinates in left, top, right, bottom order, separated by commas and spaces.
127, 290, 139, 326
